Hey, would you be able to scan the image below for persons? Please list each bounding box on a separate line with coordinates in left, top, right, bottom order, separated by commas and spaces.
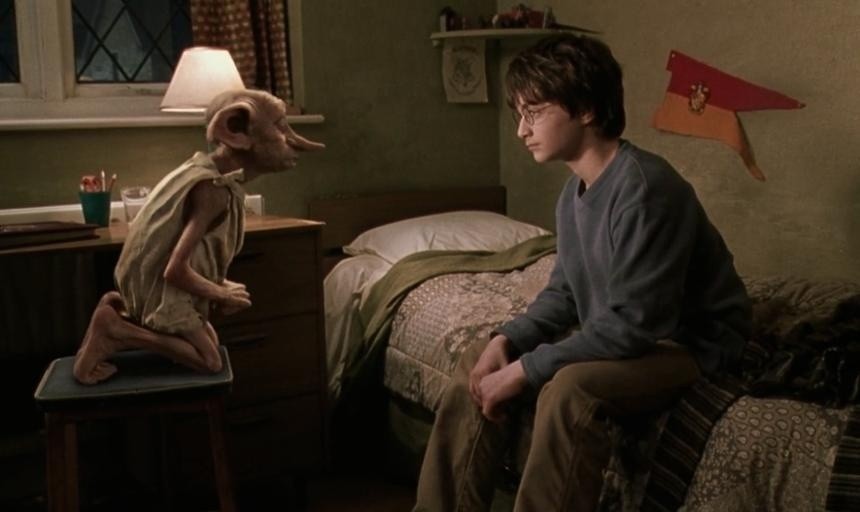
410, 30, 755, 511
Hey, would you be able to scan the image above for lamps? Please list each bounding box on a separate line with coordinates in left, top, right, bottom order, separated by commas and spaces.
157, 42, 248, 115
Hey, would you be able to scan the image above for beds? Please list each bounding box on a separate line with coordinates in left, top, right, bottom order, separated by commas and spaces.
307, 183, 859, 512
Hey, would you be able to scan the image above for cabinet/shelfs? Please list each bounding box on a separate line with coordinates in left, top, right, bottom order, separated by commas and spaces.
0, 214, 335, 512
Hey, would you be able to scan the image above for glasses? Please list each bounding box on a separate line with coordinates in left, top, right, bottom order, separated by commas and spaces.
511, 101, 559, 126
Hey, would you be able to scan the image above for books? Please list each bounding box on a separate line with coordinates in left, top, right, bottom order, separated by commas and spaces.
0, 218, 101, 252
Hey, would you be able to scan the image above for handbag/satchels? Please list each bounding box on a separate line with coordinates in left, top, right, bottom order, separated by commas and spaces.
748, 338, 856, 410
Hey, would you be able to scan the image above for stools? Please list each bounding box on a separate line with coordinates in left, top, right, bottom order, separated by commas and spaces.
34, 342, 239, 512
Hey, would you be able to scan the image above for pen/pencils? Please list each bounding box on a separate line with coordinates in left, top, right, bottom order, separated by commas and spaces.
78, 169, 117, 194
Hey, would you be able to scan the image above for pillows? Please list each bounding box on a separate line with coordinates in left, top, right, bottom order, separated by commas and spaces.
341, 208, 554, 266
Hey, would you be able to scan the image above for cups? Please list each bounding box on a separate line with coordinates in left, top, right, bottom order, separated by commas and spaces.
78, 189, 112, 229
120, 187, 150, 226
